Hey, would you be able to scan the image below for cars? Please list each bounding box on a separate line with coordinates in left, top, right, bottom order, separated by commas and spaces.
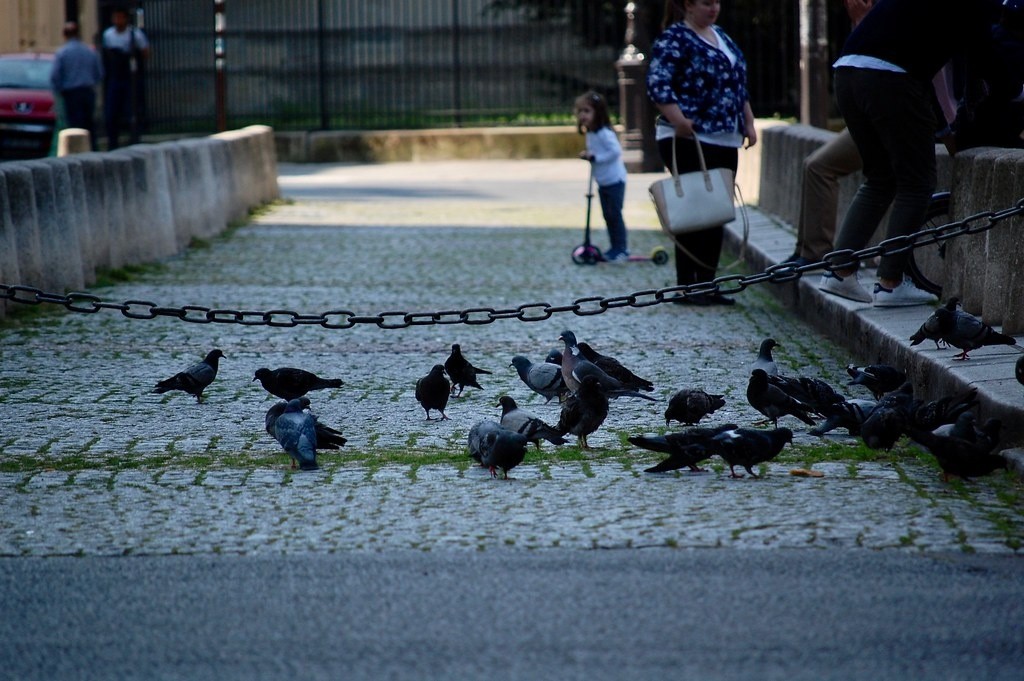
0, 51, 59, 160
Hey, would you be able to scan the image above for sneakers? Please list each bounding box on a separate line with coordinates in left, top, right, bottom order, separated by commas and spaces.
822, 265, 873, 302
672, 292, 735, 306
873, 276, 939, 306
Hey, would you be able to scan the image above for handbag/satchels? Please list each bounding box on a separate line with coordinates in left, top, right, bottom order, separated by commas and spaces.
648, 127, 736, 235
951, 99, 1024, 151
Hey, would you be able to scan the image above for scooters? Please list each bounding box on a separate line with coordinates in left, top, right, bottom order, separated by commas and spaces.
572, 153, 669, 267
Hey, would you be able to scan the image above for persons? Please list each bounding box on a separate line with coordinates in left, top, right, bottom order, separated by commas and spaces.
817, 0, 1023, 306
51, 22, 105, 154
646, 0, 757, 305
575, 90, 629, 263
101, 6, 151, 150
779, 0, 981, 275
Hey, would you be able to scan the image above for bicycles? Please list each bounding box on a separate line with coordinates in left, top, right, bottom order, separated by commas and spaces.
901, 191, 951, 302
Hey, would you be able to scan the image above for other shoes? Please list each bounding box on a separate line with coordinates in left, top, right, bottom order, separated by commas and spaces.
602, 250, 626, 266
772, 254, 832, 278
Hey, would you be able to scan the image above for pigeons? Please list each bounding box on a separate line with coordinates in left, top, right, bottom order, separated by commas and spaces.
910, 296, 1016, 361
415, 344, 493, 421
507, 331, 656, 405
467, 376, 610, 481
252, 367, 343, 403
265, 397, 348, 471
747, 337, 1009, 484
153, 349, 227, 402
626, 389, 794, 479
